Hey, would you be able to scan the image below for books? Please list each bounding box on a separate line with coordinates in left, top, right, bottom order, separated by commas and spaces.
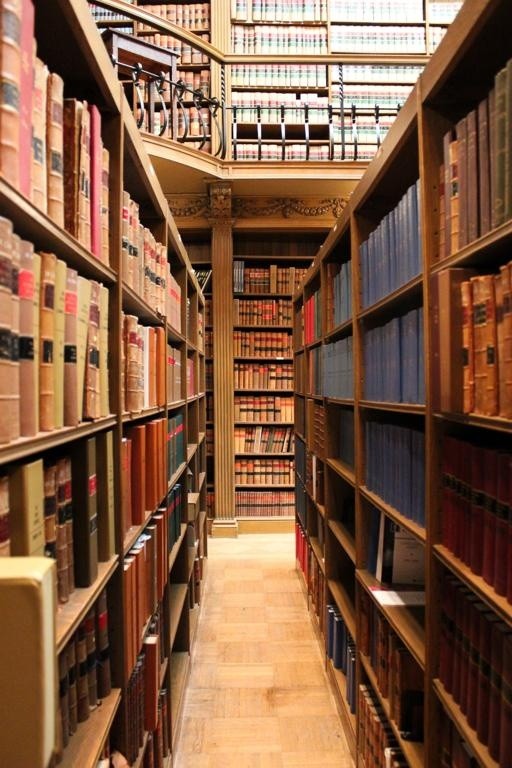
194, 260, 309, 519
0, 2, 209, 767
291, 60, 510, 767
89, 0, 467, 162
